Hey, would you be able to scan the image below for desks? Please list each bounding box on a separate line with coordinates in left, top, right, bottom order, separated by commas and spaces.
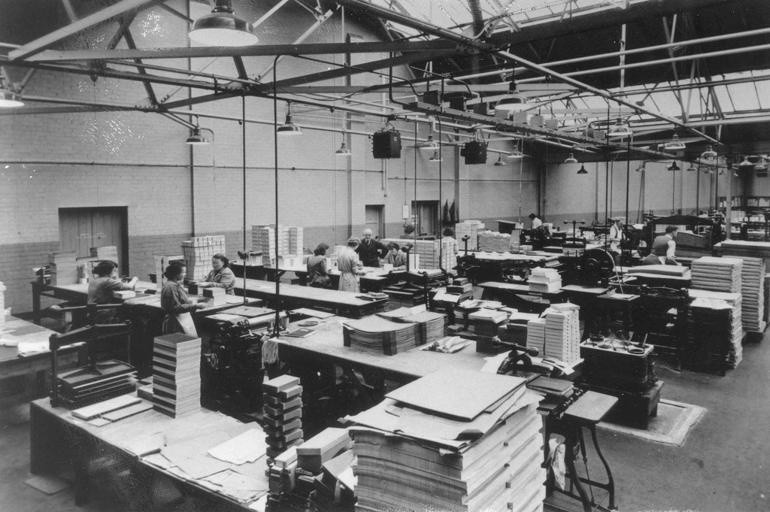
0, 253, 689, 511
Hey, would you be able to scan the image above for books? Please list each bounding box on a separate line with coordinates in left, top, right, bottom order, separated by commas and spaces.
75, 394, 268, 507
45, 223, 304, 299
455, 219, 522, 254
352, 357, 540, 512
691, 244, 766, 369
0, 265, 56, 354
342, 305, 445, 357
430, 266, 585, 375
154, 333, 202, 418
712, 197, 770, 239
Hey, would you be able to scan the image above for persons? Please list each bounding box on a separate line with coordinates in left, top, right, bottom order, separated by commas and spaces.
609, 217, 625, 252
85, 257, 133, 321
439, 228, 460, 277
643, 226, 681, 264
529, 211, 542, 229
153, 256, 201, 340
312, 229, 406, 291
205, 252, 237, 299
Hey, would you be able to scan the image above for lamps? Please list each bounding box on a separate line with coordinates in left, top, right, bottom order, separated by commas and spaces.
418, 134, 440, 151
563, 152, 579, 165
336, 134, 353, 158
576, 163, 589, 175
666, 160, 681, 172
493, 60, 531, 113
184, 0, 304, 148
493, 155, 506, 168
506, 144, 527, 160
428, 151, 445, 163
